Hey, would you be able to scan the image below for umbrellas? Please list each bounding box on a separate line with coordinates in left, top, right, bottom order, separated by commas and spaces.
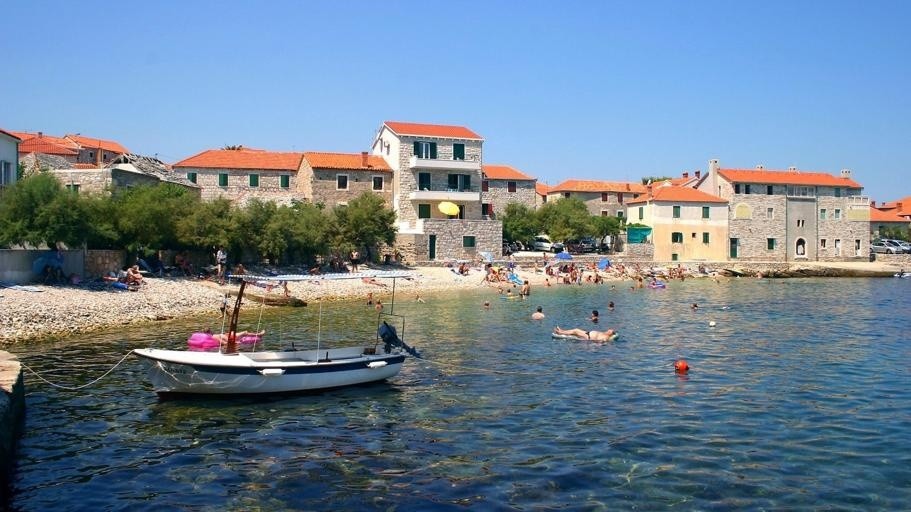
438, 201, 461, 216
31, 251, 65, 274
554, 252, 574, 262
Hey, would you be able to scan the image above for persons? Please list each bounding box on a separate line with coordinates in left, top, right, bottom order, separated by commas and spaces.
53, 264, 67, 283
484, 263, 531, 301
483, 301, 490, 308
543, 262, 688, 291
216, 291, 233, 319
383, 252, 392, 271
201, 327, 267, 346
175, 248, 194, 279
126, 264, 144, 286
350, 249, 361, 272
366, 291, 374, 305
532, 306, 545, 320
361, 277, 387, 288
40, 264, 55, 283
690, 303, 699, 313
235, 264, 246, 282
309, 264, 322, 277
459, 263, 466, 274
607, 301, 616, 312
328, 251, 350, 273
553, 325, 615, 342
215, 244, 229, 285
592, 310, 599, 322
281, 280, 292, 298
374, 299, 384, 313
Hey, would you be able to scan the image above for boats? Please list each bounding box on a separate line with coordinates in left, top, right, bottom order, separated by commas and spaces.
131, 270, 420, 400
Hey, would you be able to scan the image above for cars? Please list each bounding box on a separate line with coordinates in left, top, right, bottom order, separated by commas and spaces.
871, 238, 911, 254
502, 235, 616, 256
